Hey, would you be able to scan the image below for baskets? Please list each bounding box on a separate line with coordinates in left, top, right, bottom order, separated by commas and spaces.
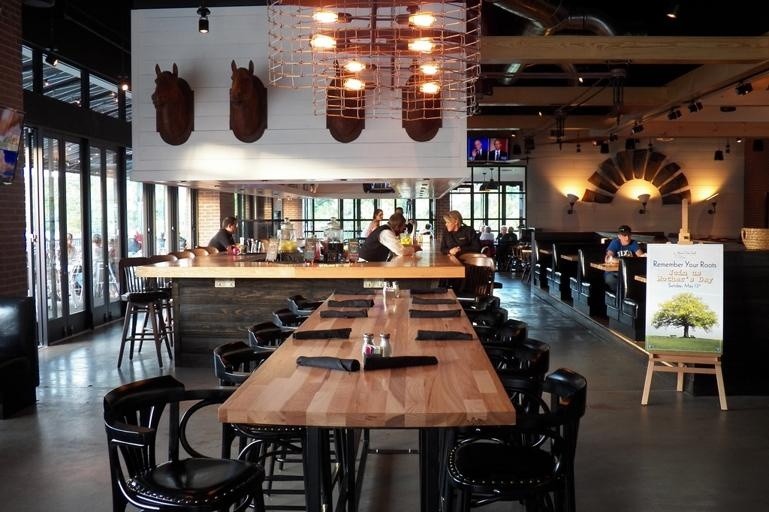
741, 227, 769, 250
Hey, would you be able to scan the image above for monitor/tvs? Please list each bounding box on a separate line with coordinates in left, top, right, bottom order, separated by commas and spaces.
1, 106, 27, 184
466, 135, 510, 166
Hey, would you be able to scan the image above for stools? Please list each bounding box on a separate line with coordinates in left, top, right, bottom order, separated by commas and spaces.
104, 373, 266, 510
248, 320, 346, 454
215, 340, 343, 497
289, 293, 323, 319
273, 307, 305, 338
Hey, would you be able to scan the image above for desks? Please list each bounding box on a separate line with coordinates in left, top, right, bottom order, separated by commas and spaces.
218, 288, 517, 512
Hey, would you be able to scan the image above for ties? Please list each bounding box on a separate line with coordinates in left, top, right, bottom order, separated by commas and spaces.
497, 150, 499, 160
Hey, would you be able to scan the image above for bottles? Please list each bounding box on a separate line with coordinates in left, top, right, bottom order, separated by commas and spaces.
380, 332, 394, 357
418, 232, 424, 243
273, 216, 348, 263
247, 238, 265, 252
382, 282, 390, 296
362, 332, 375, 359
391, 281, 401, 297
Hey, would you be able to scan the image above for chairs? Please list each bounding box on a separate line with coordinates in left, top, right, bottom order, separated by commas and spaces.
440, 290, 588, 512
115, 245, 220, 370
457, 253, 489, 293
40, 256, 127, 321
479, 241, 532, 283
1, 296, 41, 421
458, 259, 495, 301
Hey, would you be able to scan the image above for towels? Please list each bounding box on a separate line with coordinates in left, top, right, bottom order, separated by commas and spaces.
333, 288, 377, 296
409, 309, 462, 318
319, 310, 368, 318
415, 329, 473, 341
410, 287, 449, 294
412, 296, 457, 304
328, 299, 374, 308
364, 355, 439, 370
296, 355, 360, 372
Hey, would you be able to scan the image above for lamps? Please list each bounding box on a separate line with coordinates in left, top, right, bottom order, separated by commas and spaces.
638, 194, 650, 214
707, 198, 718, 214
197, 6, 211, 33
479, 168, 491, 190
266, 0, 481, 120
567, 194, 579, 214
485, 169, 497, 190
588, 79, 758, 150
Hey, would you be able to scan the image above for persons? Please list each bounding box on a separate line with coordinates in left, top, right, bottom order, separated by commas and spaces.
471, 139, 487, 160
34, 231, 187, 302
357, 213, 415, 262
638, 233, 670, 258
476, 225, 519, 271
602, 225, 646, 294
366, 207, 414, 245
489, 140, 507, 161
208, 216, 237, 252
440, 210, 481, 262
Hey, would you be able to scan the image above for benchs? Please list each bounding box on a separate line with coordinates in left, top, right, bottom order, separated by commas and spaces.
531, 226, 768, 342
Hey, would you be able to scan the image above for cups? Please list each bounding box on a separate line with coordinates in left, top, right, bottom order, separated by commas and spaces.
232, 247, 240, 255
384, 287, 397, 312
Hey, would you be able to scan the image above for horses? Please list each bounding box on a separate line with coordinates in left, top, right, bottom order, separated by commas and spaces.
152, 62, 195, 146
230, 59, 267, 142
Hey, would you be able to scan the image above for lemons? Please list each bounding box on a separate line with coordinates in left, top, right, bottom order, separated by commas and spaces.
401, 237, 411, 244
